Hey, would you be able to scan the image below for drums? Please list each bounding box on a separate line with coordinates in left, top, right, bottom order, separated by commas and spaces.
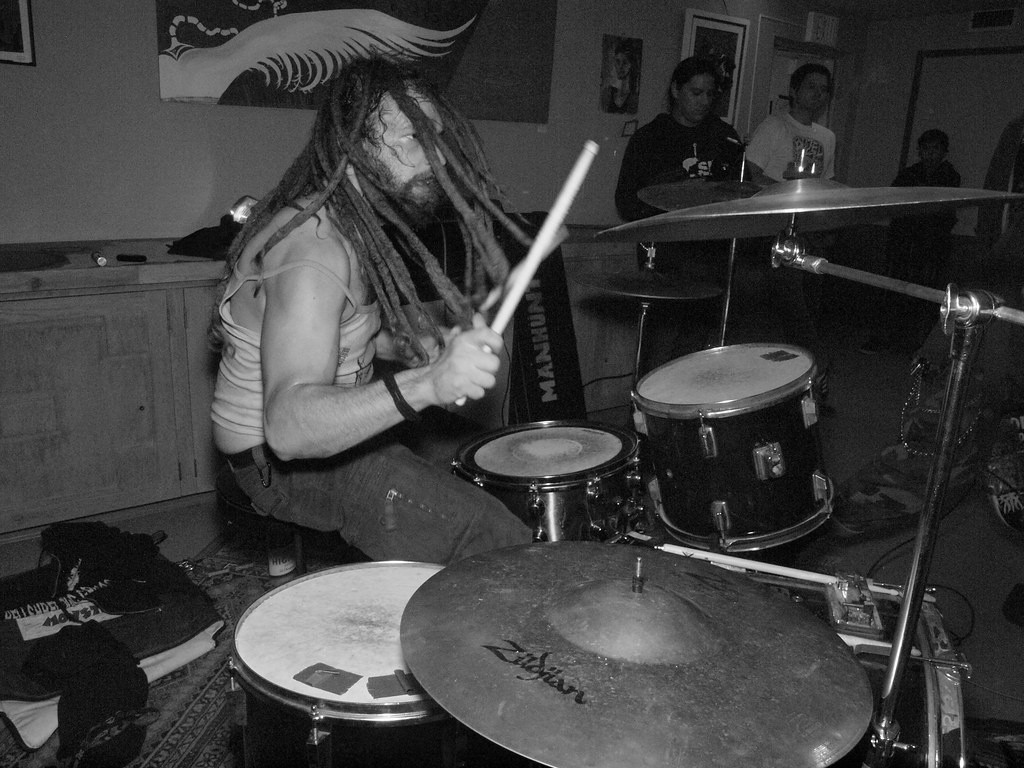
452, 415, 630, 543
224, 560, 469, 767
629, 341, 835, 554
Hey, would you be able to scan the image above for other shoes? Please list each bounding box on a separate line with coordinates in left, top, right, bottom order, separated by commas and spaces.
860, 336, 882, 355
815, 366, 836, 417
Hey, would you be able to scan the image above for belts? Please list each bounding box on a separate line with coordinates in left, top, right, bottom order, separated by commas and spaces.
225, 443, 273, 468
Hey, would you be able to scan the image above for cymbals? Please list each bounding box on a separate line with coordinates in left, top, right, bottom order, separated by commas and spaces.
399, 538, 876, 768
638, 177, 763, 213
595, 177, 1024, 244
575, 270, 723, 301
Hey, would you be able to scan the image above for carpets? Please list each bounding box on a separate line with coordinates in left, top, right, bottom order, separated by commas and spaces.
0, 528, 343, 767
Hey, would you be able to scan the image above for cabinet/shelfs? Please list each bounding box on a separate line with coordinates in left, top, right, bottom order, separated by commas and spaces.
0, 228, 646, 535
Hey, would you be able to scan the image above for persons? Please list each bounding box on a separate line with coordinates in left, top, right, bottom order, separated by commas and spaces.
615, 56, 743, 495
744, 65, 836, 418
885, 129, 963, 361
600, 39, 640, 111
209, 54, 533, 567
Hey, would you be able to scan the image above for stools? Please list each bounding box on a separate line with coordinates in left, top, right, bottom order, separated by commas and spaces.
217, 459, 332, 588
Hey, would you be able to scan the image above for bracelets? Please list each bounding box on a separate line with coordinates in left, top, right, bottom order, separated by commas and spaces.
382, 370, 423, 422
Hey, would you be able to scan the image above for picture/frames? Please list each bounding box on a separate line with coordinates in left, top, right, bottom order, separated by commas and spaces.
682, 7, 751, 134
0, 1, 39, 67
